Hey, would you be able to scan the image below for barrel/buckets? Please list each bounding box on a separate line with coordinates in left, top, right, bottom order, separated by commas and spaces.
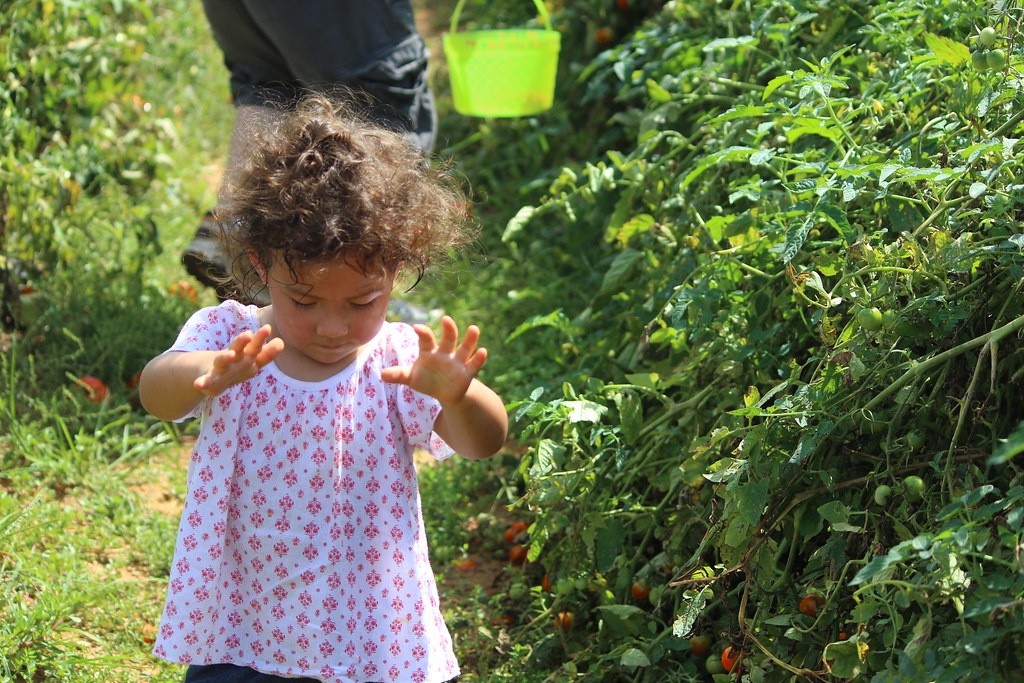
443, 0, 562, 118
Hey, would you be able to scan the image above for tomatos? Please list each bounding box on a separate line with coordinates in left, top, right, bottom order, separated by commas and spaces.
505, 303, 931, 676
73, 375, 107, 407
967, 26, 1010, 72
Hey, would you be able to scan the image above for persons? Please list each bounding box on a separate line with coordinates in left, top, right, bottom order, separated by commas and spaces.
136, 87, 509, 681
180, 0, 441, 309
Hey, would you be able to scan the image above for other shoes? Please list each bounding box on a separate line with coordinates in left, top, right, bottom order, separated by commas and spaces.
181, 208, 270, 307
